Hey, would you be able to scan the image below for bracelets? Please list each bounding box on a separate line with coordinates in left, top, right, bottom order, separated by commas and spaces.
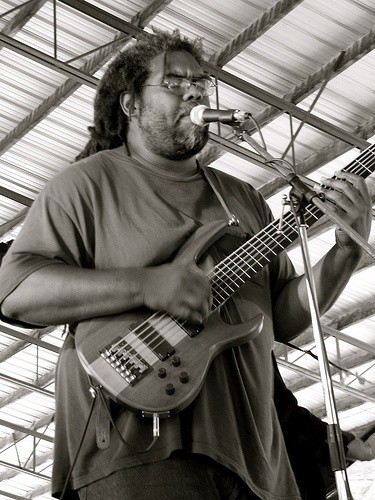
367, 443, 372, 461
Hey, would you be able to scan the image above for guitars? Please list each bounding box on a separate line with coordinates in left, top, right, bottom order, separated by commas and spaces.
75, 144, 375, 417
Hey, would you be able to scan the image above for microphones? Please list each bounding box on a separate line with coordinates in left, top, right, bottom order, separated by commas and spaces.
189, 105, 252, 126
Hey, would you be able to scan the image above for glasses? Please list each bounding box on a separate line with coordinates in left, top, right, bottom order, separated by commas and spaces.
141, 77, 217, 97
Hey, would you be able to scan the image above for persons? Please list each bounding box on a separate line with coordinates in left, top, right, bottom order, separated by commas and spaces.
0, 28, 372, 500
270, 348, 375, 500
